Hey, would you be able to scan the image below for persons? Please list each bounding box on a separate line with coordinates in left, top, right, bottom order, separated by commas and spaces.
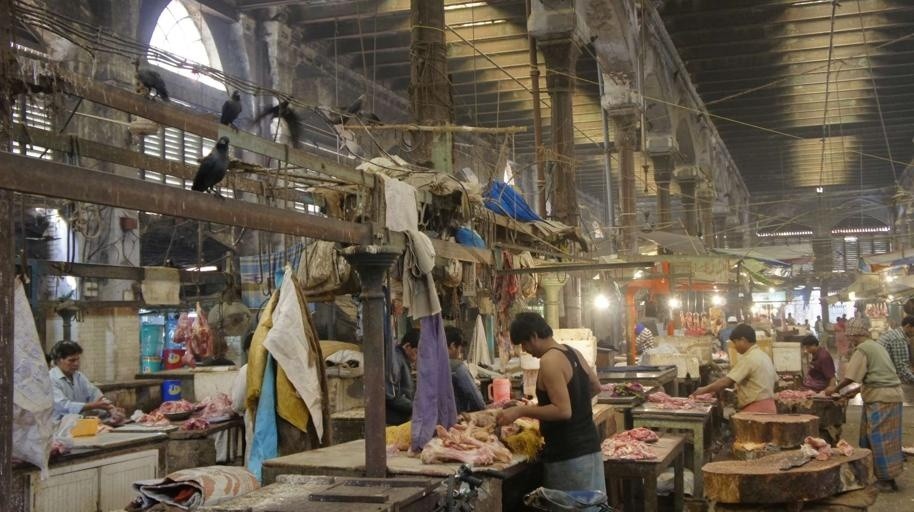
386, 328, 421, 426
693, 313, 914, 493
48, 340, 126, 431
444, 325, 487, 415
226, 333, 255, 468
496, 313, 608, 502
634, 322, 654, 355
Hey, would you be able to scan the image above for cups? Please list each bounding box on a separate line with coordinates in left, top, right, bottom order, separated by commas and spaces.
488, 377, 510, 402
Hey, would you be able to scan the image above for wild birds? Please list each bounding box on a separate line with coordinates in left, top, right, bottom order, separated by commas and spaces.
131, 58, 172, 102
250, 96, 304, 148
270, 8, 290, 23
220, 90, 242, 135
455, 108, 473, 125
192, 137, 230, 201
358, 110, 384, 127
348, 94, 367, 113
591, 35, 599, 43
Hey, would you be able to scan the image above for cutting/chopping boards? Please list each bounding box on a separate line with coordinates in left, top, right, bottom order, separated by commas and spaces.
701, 445, 873, 503
732, 394, 848, 443
108, 425, 179, 433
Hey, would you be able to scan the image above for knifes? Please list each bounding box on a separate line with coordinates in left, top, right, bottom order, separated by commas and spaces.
779, 455, 813, 469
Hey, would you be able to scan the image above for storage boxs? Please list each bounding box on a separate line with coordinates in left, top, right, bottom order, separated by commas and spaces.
727, 341, 773, 368
520, 328, 597, 370
772, 341, 802, 372
188, 366, 245, 401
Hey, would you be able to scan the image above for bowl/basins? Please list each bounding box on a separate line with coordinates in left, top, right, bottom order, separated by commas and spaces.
164, 410, 192, 420
71, 419, 101, 436
